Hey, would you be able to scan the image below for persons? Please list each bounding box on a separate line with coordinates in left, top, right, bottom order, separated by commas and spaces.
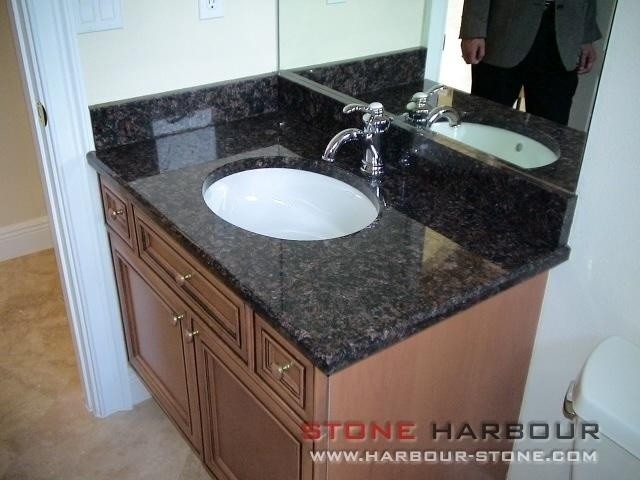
459, 0, 604, 126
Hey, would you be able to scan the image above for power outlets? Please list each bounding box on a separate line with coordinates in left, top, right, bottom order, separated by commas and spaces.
198, 0, 224, 19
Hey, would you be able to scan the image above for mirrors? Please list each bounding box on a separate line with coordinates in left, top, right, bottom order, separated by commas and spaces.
275, 0, 618, 191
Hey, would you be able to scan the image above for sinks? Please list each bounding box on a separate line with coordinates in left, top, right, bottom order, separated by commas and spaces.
321, 100, 391, 177
402, 110, 561, 171
201, 157, 381, 241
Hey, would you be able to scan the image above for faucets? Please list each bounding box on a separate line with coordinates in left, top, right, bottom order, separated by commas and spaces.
404, 84, 461, 130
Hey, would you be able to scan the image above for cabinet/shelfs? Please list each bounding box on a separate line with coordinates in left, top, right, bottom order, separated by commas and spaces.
95, 175, 550, 480
